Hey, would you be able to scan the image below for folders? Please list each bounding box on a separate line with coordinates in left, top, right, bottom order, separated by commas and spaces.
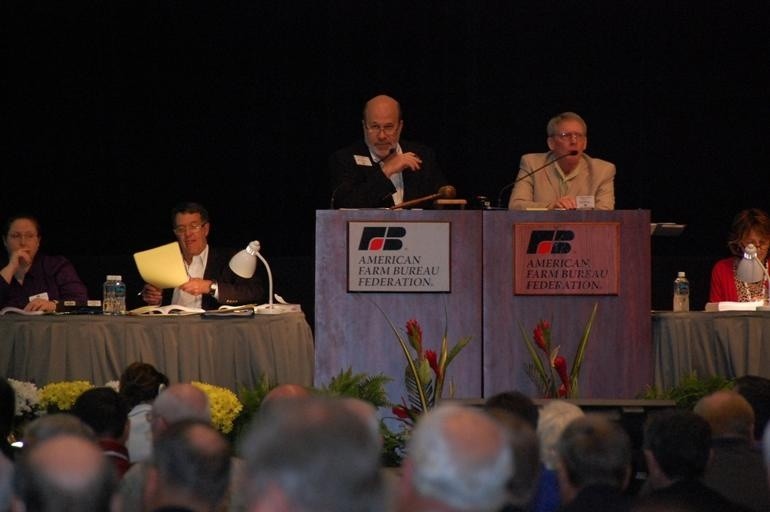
128, 304, 207, 316
1, 306, 45, 319
202, 306, 256, 321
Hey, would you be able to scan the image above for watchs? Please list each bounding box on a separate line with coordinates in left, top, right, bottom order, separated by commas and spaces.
209, 280, 216, 296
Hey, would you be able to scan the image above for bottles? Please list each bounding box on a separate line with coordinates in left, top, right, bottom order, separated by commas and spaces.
102, 275, 115, 315
115, 276, 126, 315
674, 271, 689, 312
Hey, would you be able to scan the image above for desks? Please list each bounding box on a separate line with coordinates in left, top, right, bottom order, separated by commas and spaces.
483, 210, 651, 406
650, 311, 770, 399
1, 310, 316, 396
315, 211, 482, 401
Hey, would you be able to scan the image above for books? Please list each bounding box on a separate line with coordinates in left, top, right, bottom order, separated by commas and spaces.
705, 302, 757, 312
0, 306, 44, 316
128, 305, 205, 315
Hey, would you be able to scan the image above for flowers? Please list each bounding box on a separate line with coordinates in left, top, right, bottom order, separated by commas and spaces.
38, 381, 94, 412
192, 382, 243, 435
518, 303, 598, 398
369, 300, 472, 414
8, 378, 37, 416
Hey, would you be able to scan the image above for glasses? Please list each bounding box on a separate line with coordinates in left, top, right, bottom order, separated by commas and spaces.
549, 132, 585, 142
173, 221, 206, 234
363, 120, 401, 135
737, 240, 770, 252
145, 409, 168, 429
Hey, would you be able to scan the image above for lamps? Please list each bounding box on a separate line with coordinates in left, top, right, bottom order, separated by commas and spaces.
229, 241, 282, 314
737, 244, 766, 311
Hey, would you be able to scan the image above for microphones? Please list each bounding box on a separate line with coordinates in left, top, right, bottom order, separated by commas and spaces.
486, 150, 578, 201
327, 148, 395, 207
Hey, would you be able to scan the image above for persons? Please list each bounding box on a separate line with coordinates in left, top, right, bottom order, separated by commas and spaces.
119, 385, 210, 512
25, 437, 114, 512
508, 112, 615, 210
399, 404, 513, 512
231, 397, 383, 510
119, 361, 170, 462
489, 410, 539, 512
710, 208, 770, 309
639, 410, 737, 511
540, 400, 585, 512
560, 416, 637, 512
333, 94, 442, 210
0, 377, 26, 512
694, 391, 770, 512
0, 217, 88, 313
143, 420, 230, 512
142, 202, 264, 309
15, 415, 100, 499
74, 387, 130, 476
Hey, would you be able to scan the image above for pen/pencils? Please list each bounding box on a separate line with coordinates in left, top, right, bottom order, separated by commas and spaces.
137, 288, 148, 295
